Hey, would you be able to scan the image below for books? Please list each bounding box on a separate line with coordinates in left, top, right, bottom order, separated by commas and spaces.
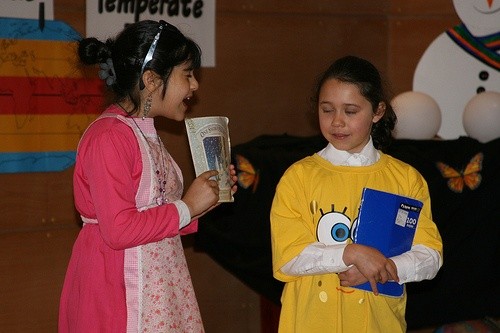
341, 187, 424, 299
184, 116, 236, 202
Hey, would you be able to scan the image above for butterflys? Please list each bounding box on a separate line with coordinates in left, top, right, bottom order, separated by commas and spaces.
436, 151, 484, 194
235, 152, 260, 194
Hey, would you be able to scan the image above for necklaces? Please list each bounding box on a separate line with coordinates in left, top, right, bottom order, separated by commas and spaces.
113, 100, 169, 206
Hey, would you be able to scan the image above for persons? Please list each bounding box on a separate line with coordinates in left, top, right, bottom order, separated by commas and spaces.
57, 20, 237, 333
270, 56, 442, 332
